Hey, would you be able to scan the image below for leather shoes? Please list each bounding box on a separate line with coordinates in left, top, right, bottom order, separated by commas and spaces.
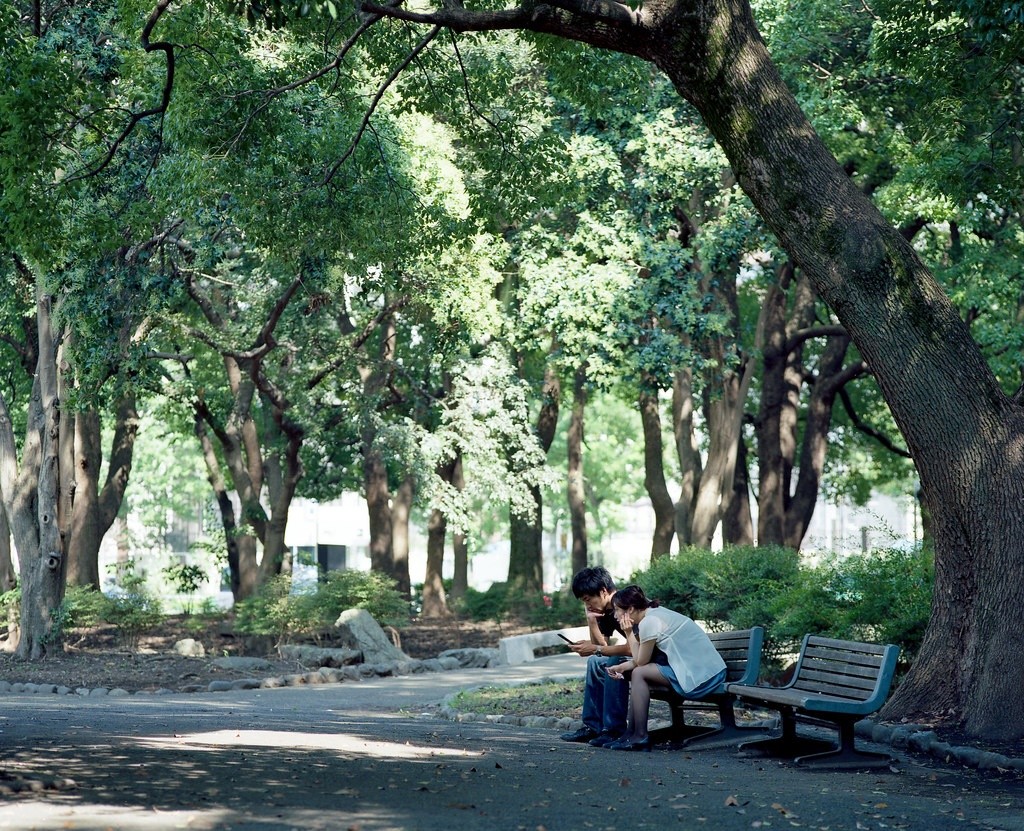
603, 736, 630, 748
588, 729, 623, 746
560, 724, 599, 742
611, 736, 653, 752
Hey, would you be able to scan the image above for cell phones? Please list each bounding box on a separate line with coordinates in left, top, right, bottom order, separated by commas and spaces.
557, 634, 574, 645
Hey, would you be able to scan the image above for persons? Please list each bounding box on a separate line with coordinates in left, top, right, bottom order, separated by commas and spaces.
603, 586, 727, 751
560, 567, 652, 747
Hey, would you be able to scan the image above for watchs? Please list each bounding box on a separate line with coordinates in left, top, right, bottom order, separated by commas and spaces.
595, 645, 602, 657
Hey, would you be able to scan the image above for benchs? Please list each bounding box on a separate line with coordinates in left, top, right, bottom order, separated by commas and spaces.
723, 633, 900, 771
629, 626, 770, 752
